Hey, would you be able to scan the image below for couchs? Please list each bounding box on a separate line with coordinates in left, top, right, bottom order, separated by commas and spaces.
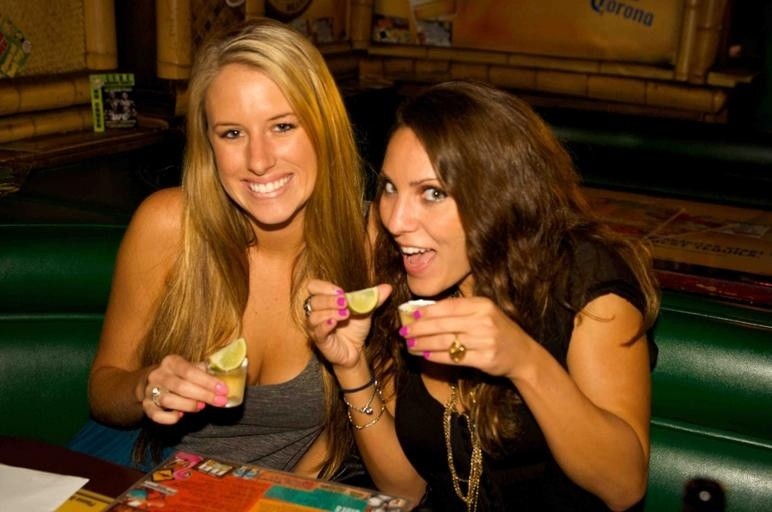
0, 219, 771, 512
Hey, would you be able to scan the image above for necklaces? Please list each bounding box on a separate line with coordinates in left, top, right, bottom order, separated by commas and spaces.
444, 366, 484, 511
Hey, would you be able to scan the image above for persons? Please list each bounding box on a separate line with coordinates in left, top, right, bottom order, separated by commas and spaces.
105, 91, 120, 121
117, 90, 137, 121
303, 79, 661, 512
72, 18, 372, 481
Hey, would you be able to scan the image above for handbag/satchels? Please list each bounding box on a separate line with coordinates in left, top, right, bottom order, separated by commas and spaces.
64, 419, 178, 475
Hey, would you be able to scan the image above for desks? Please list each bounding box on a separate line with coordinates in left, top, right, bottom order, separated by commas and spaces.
0, 432, 146, 512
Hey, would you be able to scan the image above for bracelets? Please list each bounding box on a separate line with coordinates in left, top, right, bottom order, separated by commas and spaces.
347, 396, 386, 431
338, 373, 375, 393
343, 379, 378, 415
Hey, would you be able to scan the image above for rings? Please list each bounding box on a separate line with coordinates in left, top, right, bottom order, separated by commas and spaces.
304, 294, 315, 318
151, 387, 168, 407
448, 334, 466, 362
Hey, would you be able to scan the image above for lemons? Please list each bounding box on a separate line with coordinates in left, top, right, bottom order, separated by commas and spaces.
342, 287, 378, 318
201, 337, 248, 370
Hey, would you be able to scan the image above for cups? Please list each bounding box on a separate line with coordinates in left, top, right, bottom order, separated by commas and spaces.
207, 358, 247, 408
398, 300, 438, 356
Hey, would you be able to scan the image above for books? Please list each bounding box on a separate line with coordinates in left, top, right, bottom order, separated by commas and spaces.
89, 73, 138, 133
